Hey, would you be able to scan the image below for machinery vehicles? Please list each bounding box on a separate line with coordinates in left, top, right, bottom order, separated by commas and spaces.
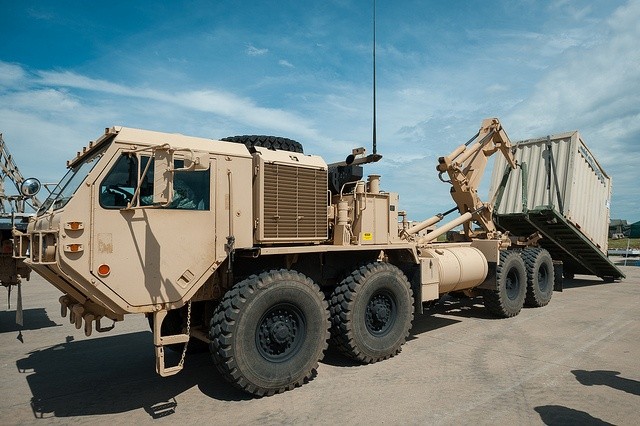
9, 0, 626, 396
1, 132, 63, 287
